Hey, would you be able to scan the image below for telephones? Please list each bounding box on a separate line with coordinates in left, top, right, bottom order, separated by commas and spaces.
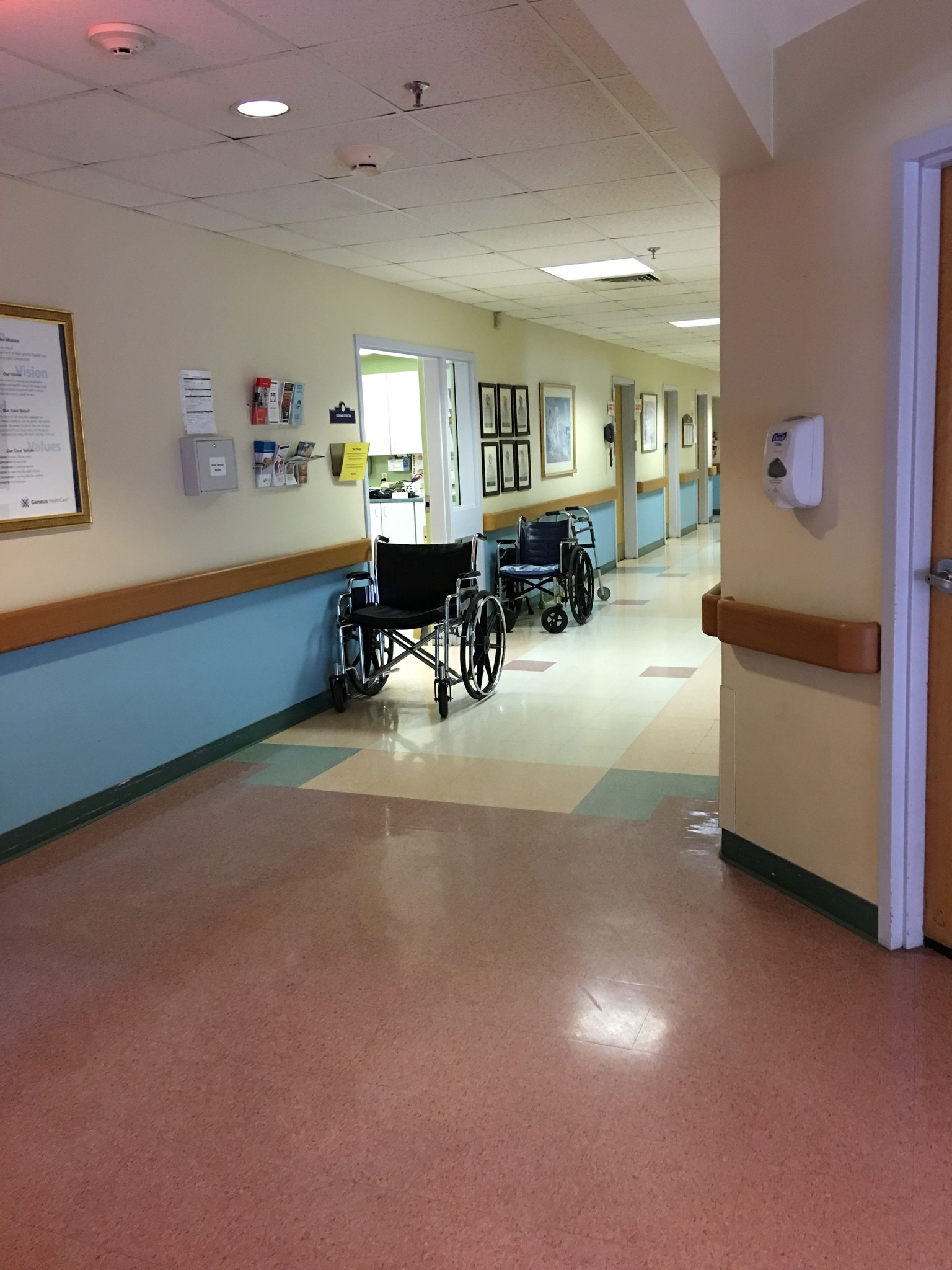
604, 423, 615, 443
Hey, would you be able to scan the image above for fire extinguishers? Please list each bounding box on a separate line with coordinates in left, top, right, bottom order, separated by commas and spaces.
712, 432, 718, 455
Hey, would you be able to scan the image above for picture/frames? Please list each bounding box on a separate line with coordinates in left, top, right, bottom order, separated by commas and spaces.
641, 393, 659, 453
481, 440, 532, 497
539, 382, 577, 478
479, 382, 530, 438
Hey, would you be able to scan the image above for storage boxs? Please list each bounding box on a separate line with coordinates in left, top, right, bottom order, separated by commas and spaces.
392, 481, 425, 499
180, 437, 239, 497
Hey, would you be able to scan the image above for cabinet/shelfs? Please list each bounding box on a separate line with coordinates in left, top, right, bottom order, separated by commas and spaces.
370, 502, 416, 552
362, 371, 419, 455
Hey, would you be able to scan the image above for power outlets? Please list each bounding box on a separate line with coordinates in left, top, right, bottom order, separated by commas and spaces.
382, 472, 387, 484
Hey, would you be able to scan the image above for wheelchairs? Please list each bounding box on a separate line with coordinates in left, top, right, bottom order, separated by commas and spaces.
493, 513, 594, 633
329, 532, 507, 717
